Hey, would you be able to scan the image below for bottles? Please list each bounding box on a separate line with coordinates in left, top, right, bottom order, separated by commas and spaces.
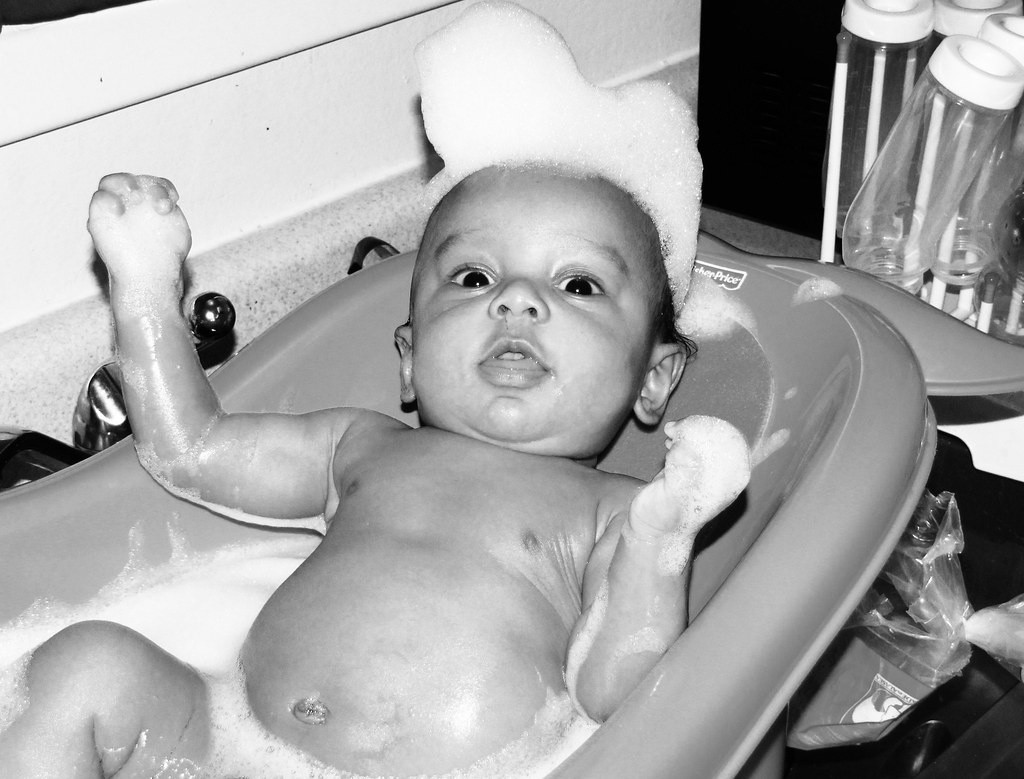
819, 0, 1024, 341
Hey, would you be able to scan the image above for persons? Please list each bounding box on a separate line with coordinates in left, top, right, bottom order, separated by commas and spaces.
0, 162, 751, 778
